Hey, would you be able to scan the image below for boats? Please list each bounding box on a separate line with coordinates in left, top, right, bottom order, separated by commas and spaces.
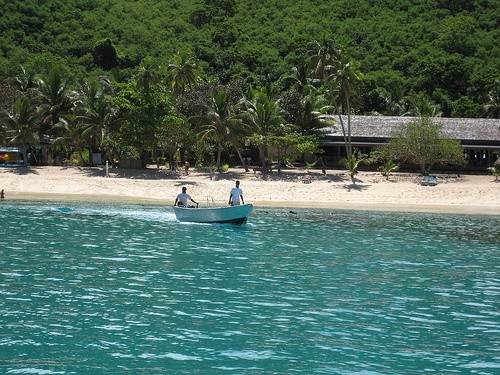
172, 203, 253, 226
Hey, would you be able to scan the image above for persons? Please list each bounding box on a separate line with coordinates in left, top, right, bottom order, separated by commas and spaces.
174, 187, 199, 208
228, 180, 244, 206
